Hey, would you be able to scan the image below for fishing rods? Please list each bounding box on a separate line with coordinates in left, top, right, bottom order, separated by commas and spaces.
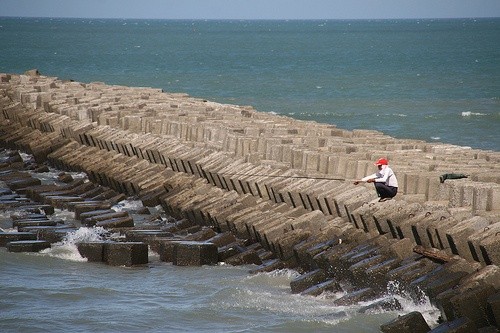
180, 171, 367, 184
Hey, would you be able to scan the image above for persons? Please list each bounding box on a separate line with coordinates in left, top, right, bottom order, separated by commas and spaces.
353, 158, 398, 203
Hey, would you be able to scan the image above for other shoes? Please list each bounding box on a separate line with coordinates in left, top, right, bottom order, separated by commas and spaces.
378, 197, 391, 202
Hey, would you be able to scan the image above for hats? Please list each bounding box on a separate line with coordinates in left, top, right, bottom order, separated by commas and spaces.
375, 157, 388, 165
